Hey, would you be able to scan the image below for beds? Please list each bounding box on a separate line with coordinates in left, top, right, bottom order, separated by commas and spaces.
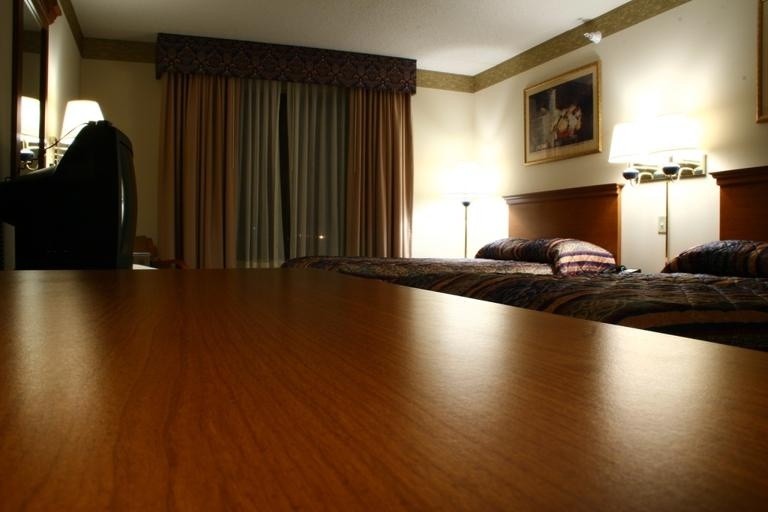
433, 165, 768, 353
285, 183, 626, 290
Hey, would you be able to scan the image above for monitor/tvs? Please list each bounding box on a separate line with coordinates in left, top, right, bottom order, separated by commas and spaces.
0, 125, 138, 270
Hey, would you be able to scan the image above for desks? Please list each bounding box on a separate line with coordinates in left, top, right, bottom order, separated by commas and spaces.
0, 264, 768, 512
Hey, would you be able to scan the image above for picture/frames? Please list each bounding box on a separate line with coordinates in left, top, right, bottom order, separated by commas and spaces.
755, 1, 768, 126
523, 57, 603, 171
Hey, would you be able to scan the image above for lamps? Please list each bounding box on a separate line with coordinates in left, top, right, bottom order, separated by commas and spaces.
51, 97, 107, 165
18, 94, 41, 163
583, 28, 601, 46
612, 124, 708, 270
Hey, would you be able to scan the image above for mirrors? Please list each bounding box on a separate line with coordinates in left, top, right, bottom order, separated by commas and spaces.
12, 1, 64, 175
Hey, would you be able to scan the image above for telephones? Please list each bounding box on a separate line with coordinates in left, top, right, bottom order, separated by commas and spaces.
607, 266, 642, 276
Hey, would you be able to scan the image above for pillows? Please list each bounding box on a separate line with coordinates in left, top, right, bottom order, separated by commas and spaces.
657, 239, 768, 279
472, 237, 617, 278
